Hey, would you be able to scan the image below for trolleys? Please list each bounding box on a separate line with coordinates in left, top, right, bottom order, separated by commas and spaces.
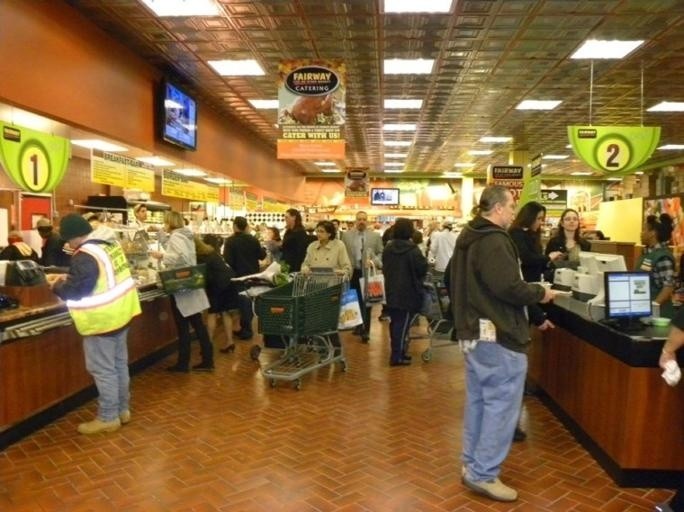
253, 268, 350, 390
403, 267, 459, 363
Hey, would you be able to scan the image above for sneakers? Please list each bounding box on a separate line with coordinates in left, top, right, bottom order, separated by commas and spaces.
513, 428, 527, 442
76, 417, 121, 434
362, 334, 370, 344
463, 471, 518, 501
118, 410, 131, 424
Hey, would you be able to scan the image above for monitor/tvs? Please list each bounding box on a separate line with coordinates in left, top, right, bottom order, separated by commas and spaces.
159, 80, 197, 151
372, 188, 399, 206
603, 271, 652, 330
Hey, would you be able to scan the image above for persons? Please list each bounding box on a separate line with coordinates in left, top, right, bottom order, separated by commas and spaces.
376, 201, 684, 446
451, 184, 557, 502
655, 482, 683, 512
3, 201, 385, 374
47, 209, 145, 436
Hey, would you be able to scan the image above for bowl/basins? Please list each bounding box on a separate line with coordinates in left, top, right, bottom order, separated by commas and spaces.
651, 317, 672, 327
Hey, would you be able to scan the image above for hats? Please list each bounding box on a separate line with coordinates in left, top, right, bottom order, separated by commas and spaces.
443, 221, 452, 230
134, 203, 146, 216
81, 212, 95, 221
60, 213, 92, 241
37, 218, 52, 228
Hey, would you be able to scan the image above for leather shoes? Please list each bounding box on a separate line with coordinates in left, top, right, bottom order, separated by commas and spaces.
391, 358, 411, 366
402, 356, 411, 360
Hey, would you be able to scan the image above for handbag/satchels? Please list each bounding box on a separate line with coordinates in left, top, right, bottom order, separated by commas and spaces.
336, 275, 363, 330
359, 260, 387, 307
158, 263, 208, 294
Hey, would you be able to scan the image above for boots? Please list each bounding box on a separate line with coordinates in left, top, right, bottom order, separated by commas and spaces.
192, 343, 213, 371
168, 346, 189, 372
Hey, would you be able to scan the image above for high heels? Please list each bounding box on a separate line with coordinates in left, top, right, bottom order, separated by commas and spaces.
220, 344, 235, 354
